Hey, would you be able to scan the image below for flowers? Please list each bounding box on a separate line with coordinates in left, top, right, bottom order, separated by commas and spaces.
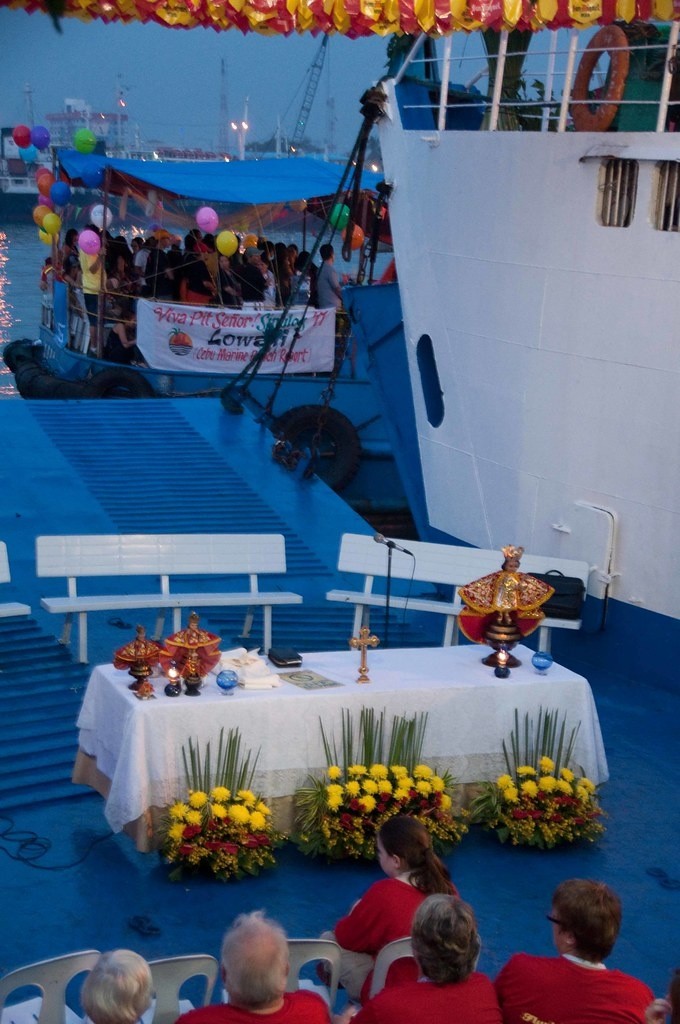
469, 707, 606, 845
160, 725, 294, 879
296, 704, 470, 859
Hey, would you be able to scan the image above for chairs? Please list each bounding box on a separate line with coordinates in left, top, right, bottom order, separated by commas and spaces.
148, 955, 220, 1024
367, 936, 485, 1000
0, 950, 102, 1023
285, 939, 343, 1003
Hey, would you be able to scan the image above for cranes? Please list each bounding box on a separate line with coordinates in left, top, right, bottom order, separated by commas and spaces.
274, 28, 338, 159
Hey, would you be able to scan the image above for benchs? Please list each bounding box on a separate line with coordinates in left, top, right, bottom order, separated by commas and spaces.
36, 533, 302, 657
0, 541, 32, 617
326, 532, 591, 656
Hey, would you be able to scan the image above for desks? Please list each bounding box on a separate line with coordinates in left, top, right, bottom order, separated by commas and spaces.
70, 640, 613, 851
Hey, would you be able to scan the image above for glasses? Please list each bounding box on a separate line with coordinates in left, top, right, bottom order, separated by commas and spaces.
543, 914, 572, 932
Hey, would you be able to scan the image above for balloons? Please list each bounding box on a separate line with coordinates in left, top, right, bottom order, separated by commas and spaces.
32, 168, 71, 246
196, 207, 219, 233
79, 230, 101, 256
74, 128, 97, 154
13, 125, 31, 148
290, 199, 307, 213
343, 224, 365, 251
19, 145, 38, 165
91, 204, 112, 230
330, 204, 350, 229
30, 126, 50, 150
217, 230, 238, 256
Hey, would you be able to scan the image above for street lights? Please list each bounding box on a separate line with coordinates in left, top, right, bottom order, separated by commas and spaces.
228, 95, 252, 159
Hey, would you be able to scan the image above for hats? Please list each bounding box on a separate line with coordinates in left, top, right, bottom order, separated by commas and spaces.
245, 247, 264, 258
194, 243, 208, 253
155, 229, 171, 240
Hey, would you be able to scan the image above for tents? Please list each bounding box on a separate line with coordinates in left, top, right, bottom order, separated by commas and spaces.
243, 233, 258, 249
50, 144, 385, 356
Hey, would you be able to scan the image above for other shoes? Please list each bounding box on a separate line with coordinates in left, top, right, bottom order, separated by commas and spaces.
136, 361, 149, 368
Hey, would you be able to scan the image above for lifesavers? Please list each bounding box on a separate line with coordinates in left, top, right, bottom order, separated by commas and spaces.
273, 402, 362, 493
85, 366, 156, 399
572, 23, 631, 132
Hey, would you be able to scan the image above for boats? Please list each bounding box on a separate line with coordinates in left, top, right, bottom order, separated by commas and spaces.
0, 70, 233, 203
5, 149, 420, 516
0, 0, 680, 625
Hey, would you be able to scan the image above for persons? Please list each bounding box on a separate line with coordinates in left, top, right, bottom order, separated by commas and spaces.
316, 244, 342, 310
40, 224, 241, 355
332, 892, 504, 1024
173, 908, 332, 1024
315, 814, 460, 1002
494, 558, 521, 624
81, 948, 153, 1024
184, 620, 203, 657
494, 880, 656, 1024
241, 241, 318, 309
134, 633, 149, 665
103, 307, 149, 368
645, 969, 680, 1024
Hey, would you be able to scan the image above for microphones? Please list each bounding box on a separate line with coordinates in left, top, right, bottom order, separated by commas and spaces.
374, 533, 414, 558
